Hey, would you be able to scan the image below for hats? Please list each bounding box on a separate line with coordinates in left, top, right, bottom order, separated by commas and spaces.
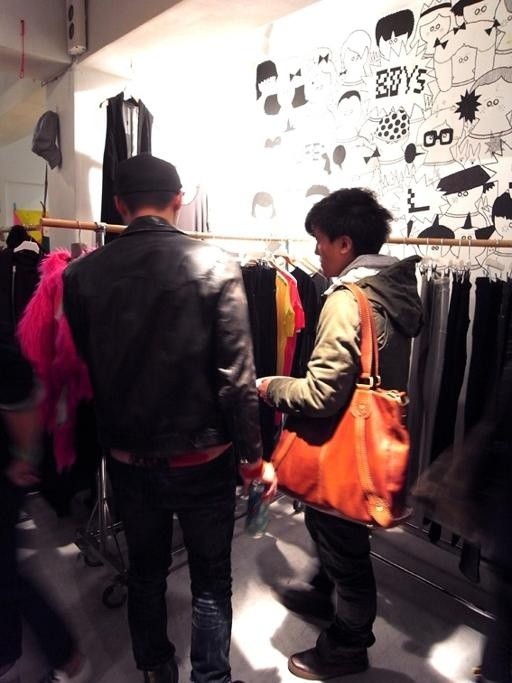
116, 155, 183, 196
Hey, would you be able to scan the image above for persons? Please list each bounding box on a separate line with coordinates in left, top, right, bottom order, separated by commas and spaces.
63, 152, 278, 682
257, 186, 425, 682
0, 263, 46, 682
409, 360, 511, 681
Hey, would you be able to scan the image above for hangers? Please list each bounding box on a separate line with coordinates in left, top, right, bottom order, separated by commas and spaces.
244, 253, 318, 277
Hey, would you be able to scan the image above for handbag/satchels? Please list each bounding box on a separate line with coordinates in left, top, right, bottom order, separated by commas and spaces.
268, 281, 417, 533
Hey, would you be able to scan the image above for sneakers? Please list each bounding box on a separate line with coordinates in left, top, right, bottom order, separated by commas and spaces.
280, 585, 336, 621
288, 644, 369, 681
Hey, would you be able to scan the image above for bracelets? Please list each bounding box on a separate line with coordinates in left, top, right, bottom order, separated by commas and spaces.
8, 445, 46, 467
